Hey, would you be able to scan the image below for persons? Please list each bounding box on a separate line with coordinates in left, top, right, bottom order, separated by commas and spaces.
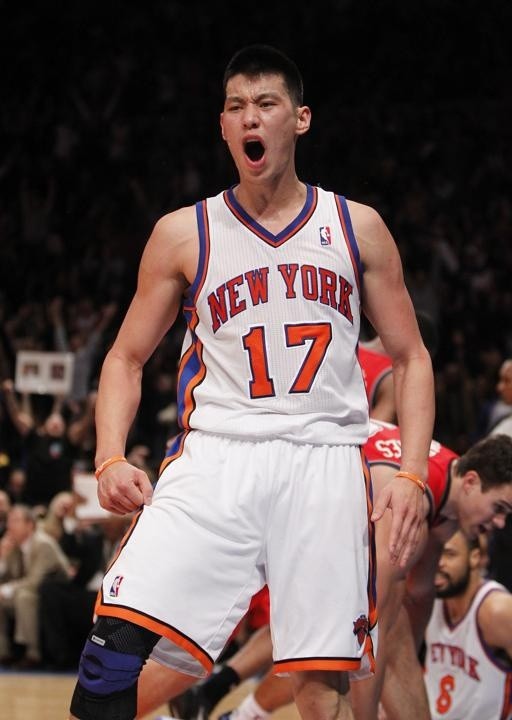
0, 188, 511, 719
64, 40, 440, 718
